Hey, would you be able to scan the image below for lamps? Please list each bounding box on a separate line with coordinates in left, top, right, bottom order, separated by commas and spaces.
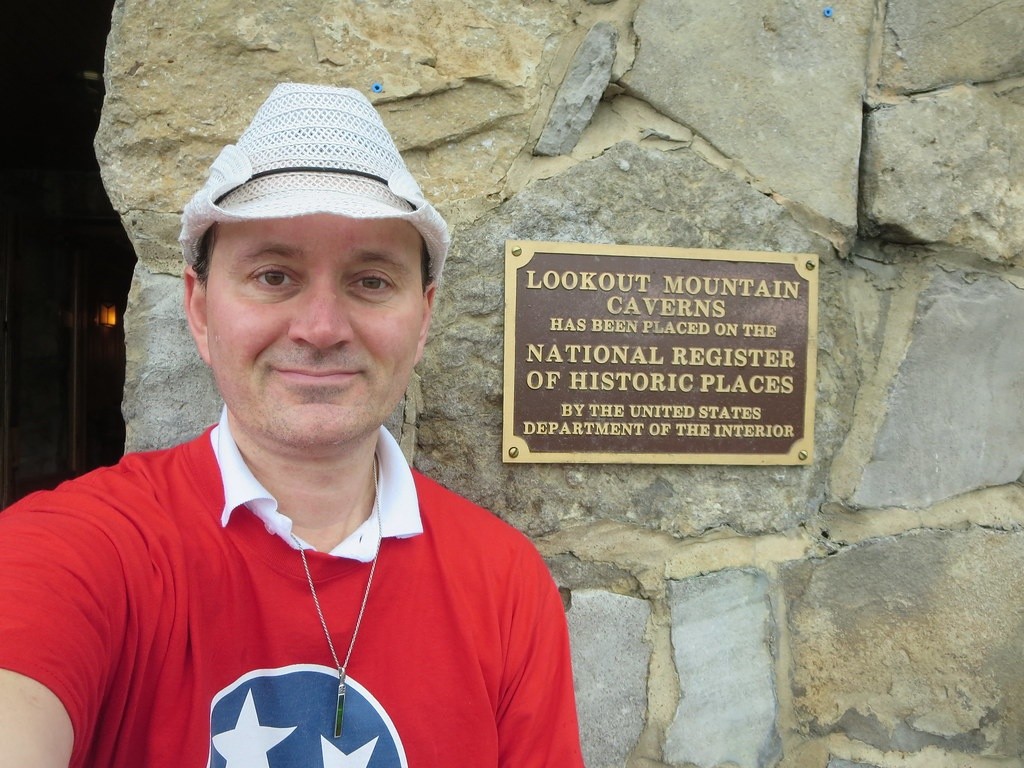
99, 300, 117, 328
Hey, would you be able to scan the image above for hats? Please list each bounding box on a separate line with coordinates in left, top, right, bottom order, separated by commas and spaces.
177, 83, 450, 286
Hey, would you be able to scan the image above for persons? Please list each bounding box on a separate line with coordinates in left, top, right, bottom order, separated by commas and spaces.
1, 83, 586, 768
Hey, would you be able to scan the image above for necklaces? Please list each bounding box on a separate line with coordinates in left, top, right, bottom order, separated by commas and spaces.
291, 458, 382, 738
